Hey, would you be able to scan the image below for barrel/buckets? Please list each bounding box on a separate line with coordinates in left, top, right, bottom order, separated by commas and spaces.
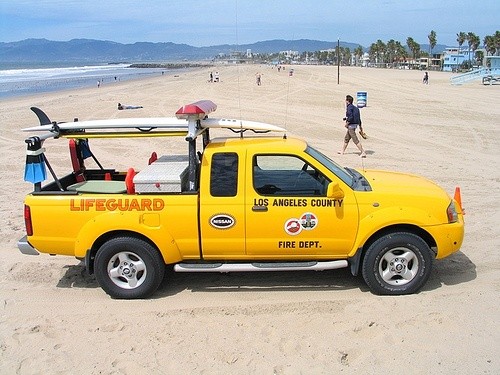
357, 92, 367, 107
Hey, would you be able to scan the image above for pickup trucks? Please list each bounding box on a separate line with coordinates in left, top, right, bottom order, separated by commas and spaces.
18, 99, 465, 300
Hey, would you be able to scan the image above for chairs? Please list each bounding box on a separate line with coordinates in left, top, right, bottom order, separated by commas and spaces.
148, 152, 158, 165
125, 168, 138, 195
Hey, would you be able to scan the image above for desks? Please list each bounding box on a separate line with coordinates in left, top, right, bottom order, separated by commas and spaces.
133, 155, 200, 195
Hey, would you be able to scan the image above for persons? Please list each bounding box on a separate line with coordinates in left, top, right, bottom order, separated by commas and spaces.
477, 56, 481, 69
97, 71, 165, 88
118, 103, 143, 110
256, 64, 294, 85
209, 72, 220, 83
485, 59, 491, 74
423, 72, 428, 84
337, 95, 366, 157
